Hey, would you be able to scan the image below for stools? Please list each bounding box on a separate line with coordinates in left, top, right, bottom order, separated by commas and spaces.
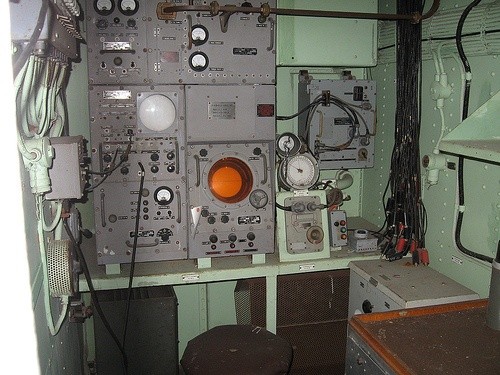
178, 325, 293, 375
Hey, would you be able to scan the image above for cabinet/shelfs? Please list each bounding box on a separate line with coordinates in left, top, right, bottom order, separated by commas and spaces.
343, 297, 500, 375
343, 259, 480, 321
